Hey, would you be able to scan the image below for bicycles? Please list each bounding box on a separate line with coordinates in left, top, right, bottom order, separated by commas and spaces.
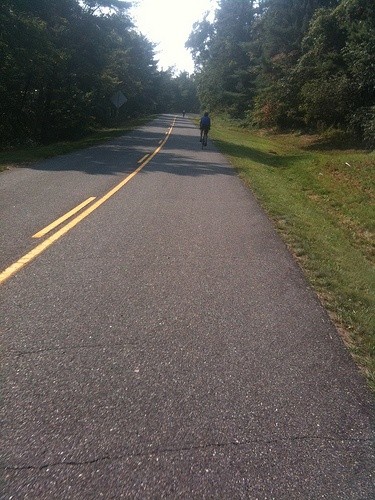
183, 112, 185, 117
199, 128, 210, 151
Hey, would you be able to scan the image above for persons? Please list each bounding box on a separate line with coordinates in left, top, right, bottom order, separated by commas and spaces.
198, 111, 209, 143
183, 109, 185, 117
